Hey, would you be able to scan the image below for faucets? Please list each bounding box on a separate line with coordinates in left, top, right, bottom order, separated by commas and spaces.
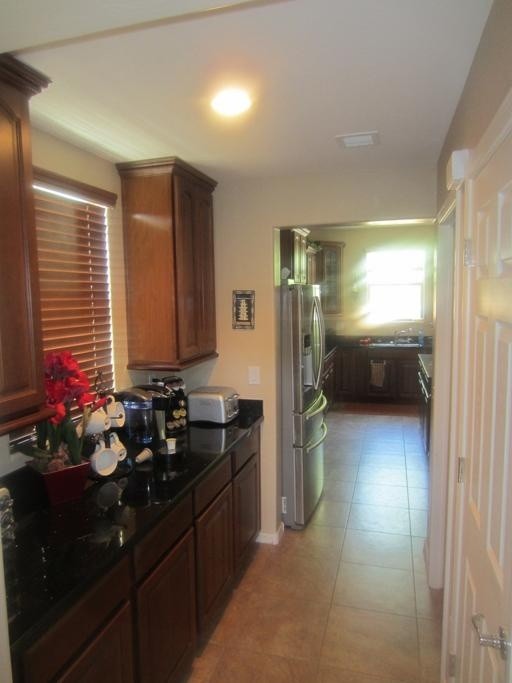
393, 327, 411, 344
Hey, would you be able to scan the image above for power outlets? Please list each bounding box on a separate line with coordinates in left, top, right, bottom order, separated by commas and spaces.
149, 375, 156, 384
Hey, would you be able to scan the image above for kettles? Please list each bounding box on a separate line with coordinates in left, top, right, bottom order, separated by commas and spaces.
134, 384, 172, 455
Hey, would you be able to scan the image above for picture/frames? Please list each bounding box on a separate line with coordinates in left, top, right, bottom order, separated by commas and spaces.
233, 290, 255, 329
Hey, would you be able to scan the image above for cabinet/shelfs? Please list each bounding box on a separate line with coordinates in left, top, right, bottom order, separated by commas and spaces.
19, 426, 261, 683
115, 157, 219, 372
0, 53, 55, 436
338, 349, 416, 402
280, 228, 345, 317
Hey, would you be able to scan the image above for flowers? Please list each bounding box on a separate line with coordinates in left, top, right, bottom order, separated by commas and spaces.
13, 351, 106, 473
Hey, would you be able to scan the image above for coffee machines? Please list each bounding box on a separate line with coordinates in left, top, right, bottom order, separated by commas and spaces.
113, 387, 160, 458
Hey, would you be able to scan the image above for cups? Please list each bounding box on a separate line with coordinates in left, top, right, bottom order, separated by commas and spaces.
135, 447, 153, 464
165, 437, 177, 455
76, 394, 128, 476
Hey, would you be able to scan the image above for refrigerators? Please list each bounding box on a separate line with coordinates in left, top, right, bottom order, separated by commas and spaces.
280, 281, 328, 530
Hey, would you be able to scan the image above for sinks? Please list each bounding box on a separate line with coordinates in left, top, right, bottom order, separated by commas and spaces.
370, 341, 420, 348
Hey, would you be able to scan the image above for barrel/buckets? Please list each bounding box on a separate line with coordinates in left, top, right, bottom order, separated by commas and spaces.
124, 401, 155, 443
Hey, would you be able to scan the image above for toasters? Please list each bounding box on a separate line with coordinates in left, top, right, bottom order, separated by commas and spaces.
187, 385, 241, 427
